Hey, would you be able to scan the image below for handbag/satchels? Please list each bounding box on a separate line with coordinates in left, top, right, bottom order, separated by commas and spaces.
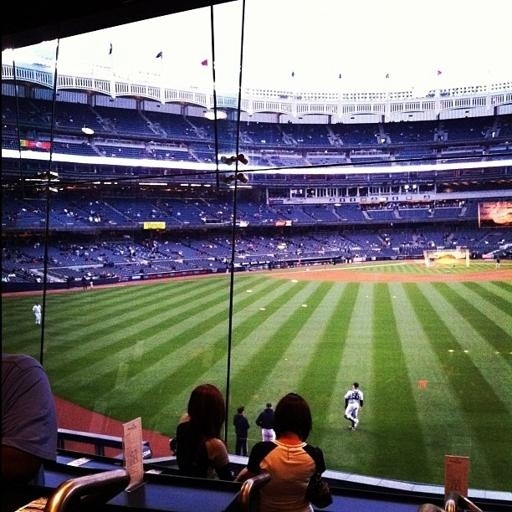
308, 477, 333, 509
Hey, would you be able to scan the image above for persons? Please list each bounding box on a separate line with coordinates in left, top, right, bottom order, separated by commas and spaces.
1, 353, 57, 512
234, 406, 250, 456
232, 392, 326, 512
256, 403, 277, 442
1, 196, 512, 289
32, 303, 43, 327
344, 383, 364, 431
176, 383, 233, 481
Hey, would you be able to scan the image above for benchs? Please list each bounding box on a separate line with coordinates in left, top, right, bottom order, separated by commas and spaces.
29, 443, 440, 512
0, 96, 512, 286
